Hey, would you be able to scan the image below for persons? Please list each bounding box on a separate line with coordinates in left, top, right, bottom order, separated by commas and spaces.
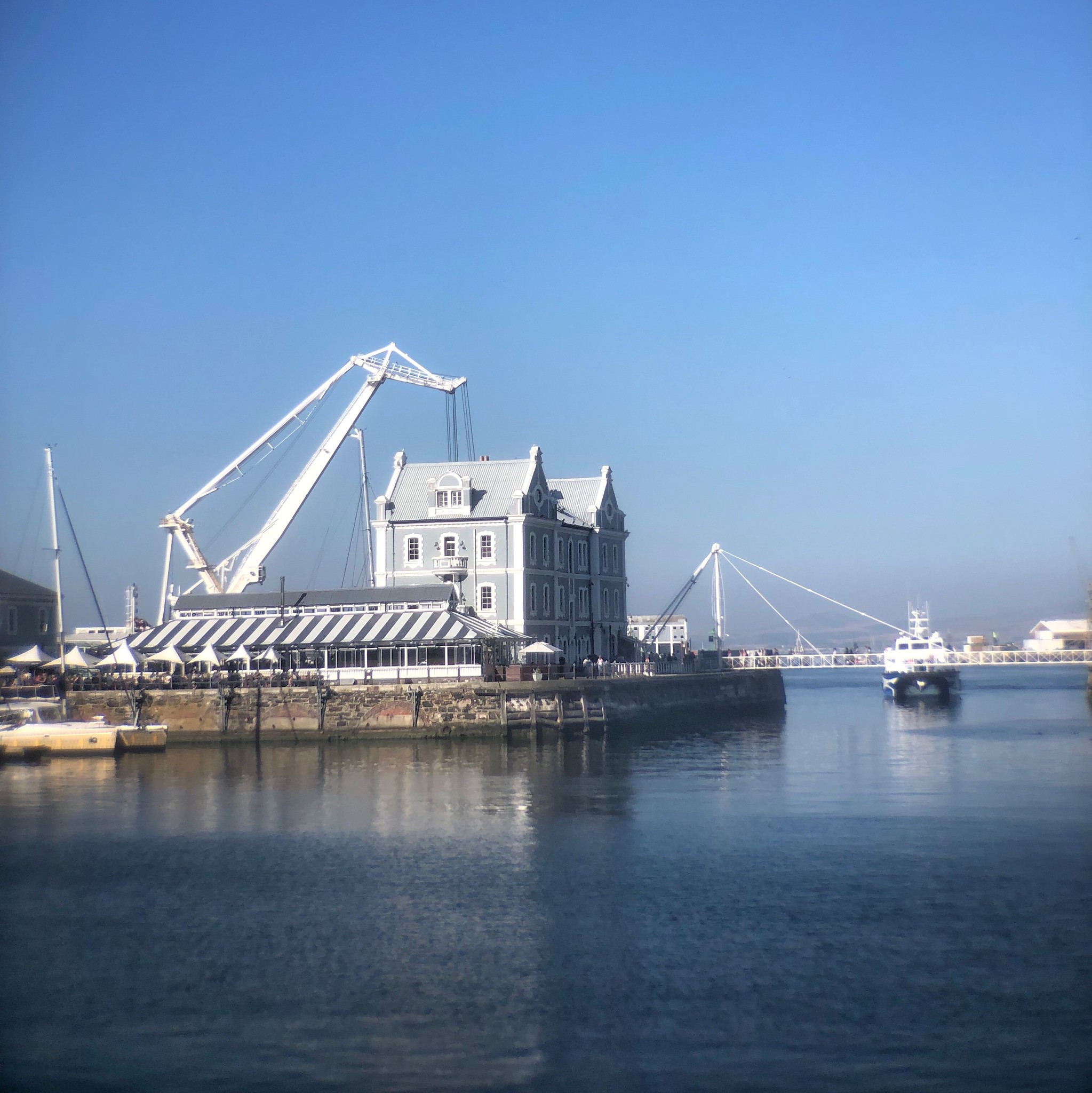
572, 644, 887, 680
0, 654, 358, 691
946, 642, 952, 651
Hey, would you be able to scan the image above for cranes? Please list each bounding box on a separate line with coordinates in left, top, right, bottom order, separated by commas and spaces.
155, 340, 469, 628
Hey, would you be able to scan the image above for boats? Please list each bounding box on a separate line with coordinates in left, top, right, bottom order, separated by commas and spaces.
882, 599, 961, 703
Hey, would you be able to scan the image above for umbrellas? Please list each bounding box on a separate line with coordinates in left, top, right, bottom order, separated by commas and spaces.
519, 641, 564, 666
93, 640, 148, 680
6, 644, 58, 681
41, 644, 103, 681
145, 642, 194, 689
187, 642, 228, 688
254, 645, 288, 689
225, 643, 257, 680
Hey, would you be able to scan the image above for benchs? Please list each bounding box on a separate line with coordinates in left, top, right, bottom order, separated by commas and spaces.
73, 678, 319, 691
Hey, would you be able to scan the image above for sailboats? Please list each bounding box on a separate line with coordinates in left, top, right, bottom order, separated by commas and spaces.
0, 447, 168, 732
